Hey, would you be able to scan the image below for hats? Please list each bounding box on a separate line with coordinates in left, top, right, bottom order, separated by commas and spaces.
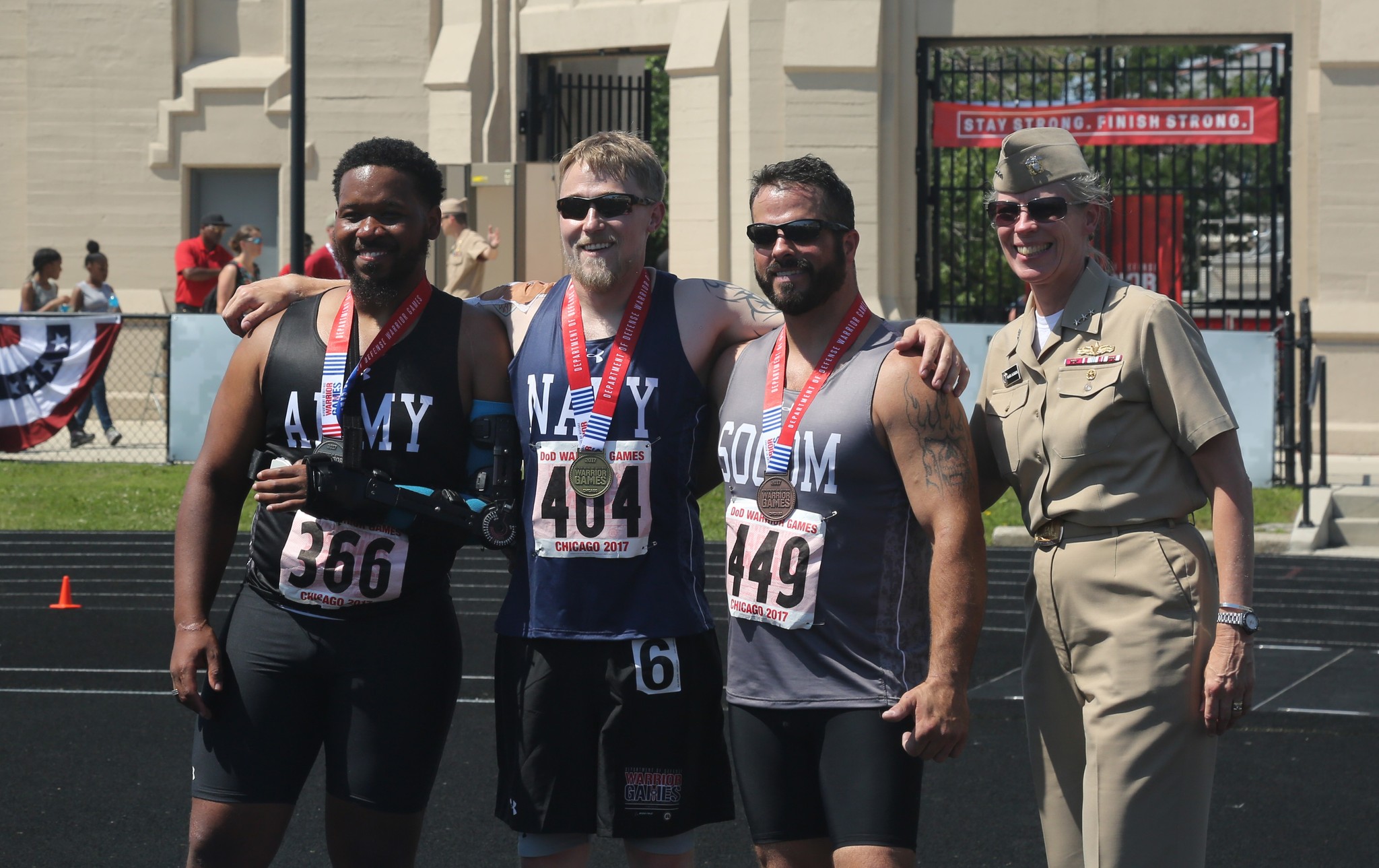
326, 213, 336, 227
992, 127, 1090, 194
200, 214, 233, 227
440, 197, 469, 213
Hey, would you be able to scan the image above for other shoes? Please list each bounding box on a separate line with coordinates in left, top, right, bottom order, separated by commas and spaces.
69, 430, 95, 448
106, 426, 122, 446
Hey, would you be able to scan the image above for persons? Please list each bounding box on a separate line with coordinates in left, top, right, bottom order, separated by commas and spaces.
216, 225, 264, 314
278, 231, 314, 277
175, 213, 234, 313
969, 129, 1258, 868
304, 212, 349, 281
169, 131, 986, 868
438, 198, 500, 300
19, 240, 123, 448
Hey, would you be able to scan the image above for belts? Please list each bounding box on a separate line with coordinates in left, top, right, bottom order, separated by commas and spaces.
1033, 512, 1188, 547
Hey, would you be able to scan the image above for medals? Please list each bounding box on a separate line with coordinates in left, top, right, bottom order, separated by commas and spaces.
568, 448, 613, 499
757, 471, 796, 522
312, 435, 343, 464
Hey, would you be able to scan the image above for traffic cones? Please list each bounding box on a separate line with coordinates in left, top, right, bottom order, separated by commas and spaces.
50, 576, 82, 610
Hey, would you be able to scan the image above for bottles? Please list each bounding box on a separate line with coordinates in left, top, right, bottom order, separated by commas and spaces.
59, 303, 70, 313
107, 293, 119, 312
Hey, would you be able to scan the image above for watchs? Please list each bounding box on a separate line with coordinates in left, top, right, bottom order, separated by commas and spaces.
1216, 611, 1260, 635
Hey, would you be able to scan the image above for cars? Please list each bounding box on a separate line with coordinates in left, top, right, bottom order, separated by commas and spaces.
1198, 218, 1259, 255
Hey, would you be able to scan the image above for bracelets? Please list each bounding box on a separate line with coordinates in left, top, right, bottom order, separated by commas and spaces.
483, 241, 498, 249
1219, 602, 1254, 612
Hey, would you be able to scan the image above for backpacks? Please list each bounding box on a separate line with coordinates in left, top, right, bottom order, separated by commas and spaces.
201, 259, 259, 314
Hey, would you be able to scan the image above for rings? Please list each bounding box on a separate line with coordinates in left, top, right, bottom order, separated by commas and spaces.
1232, 701, 1243, 711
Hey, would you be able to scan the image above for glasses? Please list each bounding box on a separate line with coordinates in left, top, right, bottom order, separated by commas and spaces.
557, 191, 655, 220
207, 224, 227, 234
746, 218, 850, 247
245, 238, 261, 244
986, 196, 1090, 226
